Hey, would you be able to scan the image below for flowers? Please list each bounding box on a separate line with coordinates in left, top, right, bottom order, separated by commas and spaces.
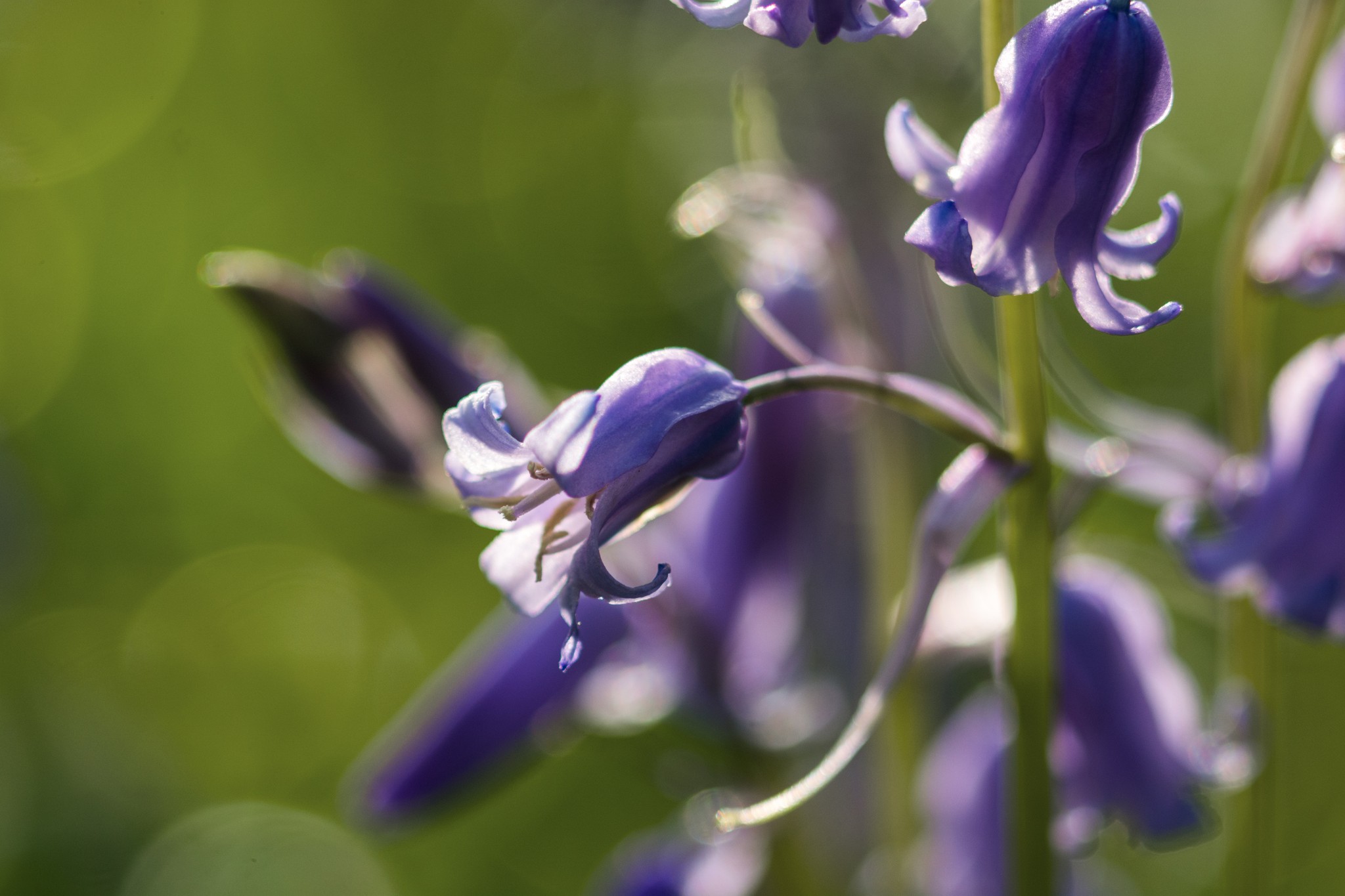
200, 0, 1345, 896
